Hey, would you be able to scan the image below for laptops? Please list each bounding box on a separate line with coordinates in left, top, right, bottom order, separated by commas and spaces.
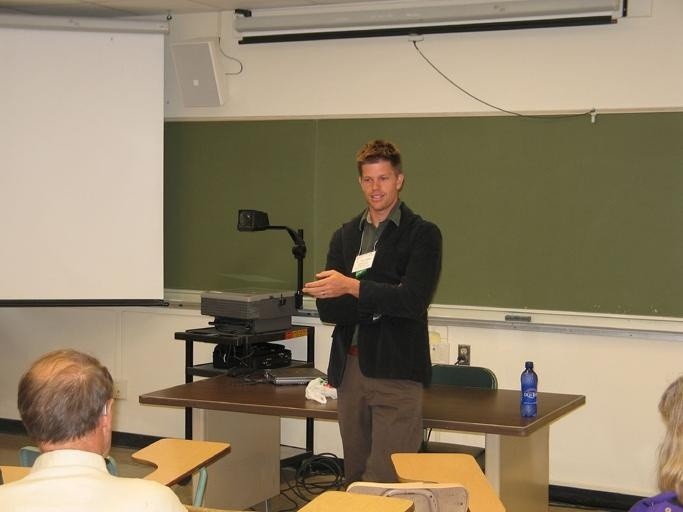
263, 367, 329, 386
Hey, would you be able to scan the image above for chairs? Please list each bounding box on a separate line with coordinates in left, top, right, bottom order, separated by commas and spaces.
419, 365, 497, 471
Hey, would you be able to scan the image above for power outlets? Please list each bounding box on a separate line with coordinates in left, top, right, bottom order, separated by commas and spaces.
458, 344, 470, 365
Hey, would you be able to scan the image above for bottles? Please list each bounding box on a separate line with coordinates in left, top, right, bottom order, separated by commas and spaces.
519, 361, 538, 418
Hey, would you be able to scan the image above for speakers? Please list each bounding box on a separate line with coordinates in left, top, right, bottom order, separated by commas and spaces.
170, 36, 227, 111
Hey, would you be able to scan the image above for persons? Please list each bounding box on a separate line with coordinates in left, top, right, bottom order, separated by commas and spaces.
301, 138, 444, 491
628, 375, 683, 512
0, 347, 190, 512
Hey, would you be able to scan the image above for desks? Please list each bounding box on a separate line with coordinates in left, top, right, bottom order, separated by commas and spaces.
139, 376, 586, 512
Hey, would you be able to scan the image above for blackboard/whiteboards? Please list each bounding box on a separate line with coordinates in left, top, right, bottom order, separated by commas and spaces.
164, 107, 683, 333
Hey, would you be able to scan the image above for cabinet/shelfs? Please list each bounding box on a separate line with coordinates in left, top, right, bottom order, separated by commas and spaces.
175, 325, 316, 469
